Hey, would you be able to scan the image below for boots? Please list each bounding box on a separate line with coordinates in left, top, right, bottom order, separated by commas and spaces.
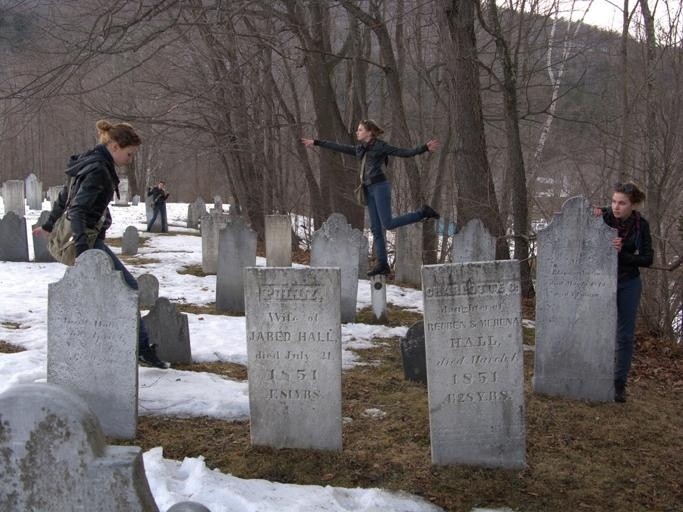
615, 383, 624, 402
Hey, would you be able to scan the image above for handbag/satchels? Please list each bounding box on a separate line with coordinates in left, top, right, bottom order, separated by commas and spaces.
353, 183, 368, 206
150, 202, 156, 208
47, 210, 97, 267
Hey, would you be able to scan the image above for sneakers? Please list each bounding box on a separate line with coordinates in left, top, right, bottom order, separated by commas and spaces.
423, 204, 440, 219
139, 351, 168, 369
367, 263, 390, 276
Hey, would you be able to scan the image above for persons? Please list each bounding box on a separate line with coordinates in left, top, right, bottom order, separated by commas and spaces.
301, 119, 440, 276
146, 181, 170, 233
32, 120, 169, 369
592, 182, 654, 404
